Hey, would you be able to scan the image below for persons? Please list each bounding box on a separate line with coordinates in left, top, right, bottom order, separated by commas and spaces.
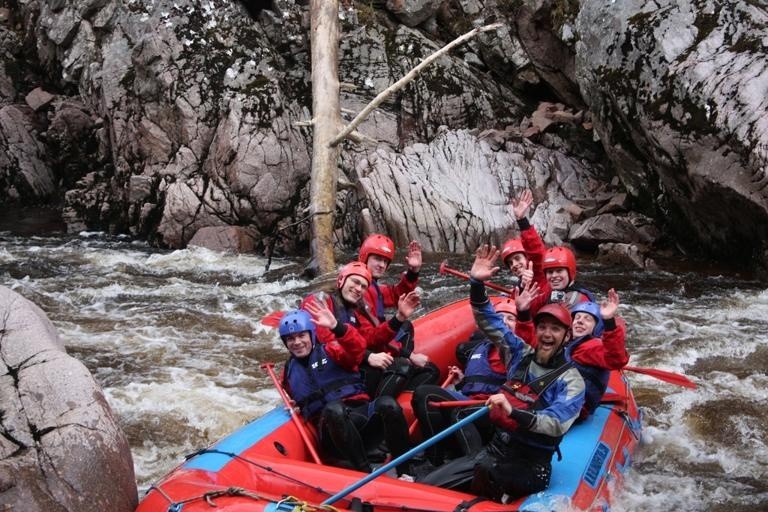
300, 260, 421, 462
453, 189, 553, 373
356, 233, 442, 392
408, 280, 542, 468
560, 286, 631, 427
277, 298, 420, 482
420, 241, 586, 505
543, 244, 595, 308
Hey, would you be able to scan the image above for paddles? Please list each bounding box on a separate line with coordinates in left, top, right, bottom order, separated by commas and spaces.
623, 365, 697, 391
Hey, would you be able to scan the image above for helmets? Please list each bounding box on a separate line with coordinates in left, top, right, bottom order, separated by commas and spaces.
278, 310, 317, 351
501, 238, 526, 269
571, 301, 605, 338
491, 297, 518, 316
336, 260, 372, 290
533, 303, 573, 328
542, 246, 576, 283
358, 234, 395, 271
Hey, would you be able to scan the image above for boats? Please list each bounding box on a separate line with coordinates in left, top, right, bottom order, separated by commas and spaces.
126, 289, 647, 512
